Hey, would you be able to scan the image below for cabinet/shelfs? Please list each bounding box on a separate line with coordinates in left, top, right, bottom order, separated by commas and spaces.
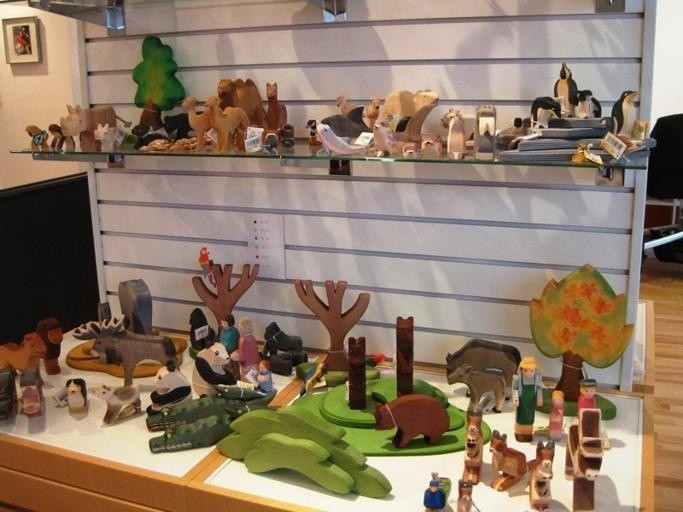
0, 135, 658, 511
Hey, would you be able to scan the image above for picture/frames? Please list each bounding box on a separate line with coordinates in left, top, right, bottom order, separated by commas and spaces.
1, 15, 42, 67
